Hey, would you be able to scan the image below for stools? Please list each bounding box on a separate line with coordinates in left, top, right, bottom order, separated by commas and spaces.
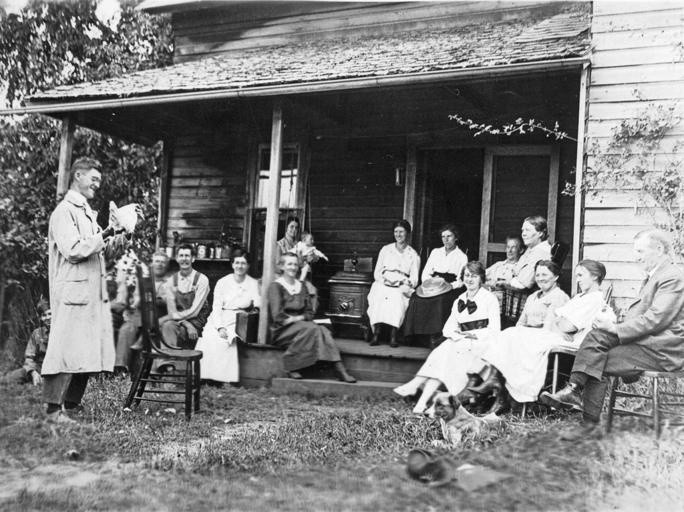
606, 371, 683, 439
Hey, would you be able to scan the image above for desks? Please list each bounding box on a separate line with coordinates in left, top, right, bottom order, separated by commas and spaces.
166, 259, 235, 313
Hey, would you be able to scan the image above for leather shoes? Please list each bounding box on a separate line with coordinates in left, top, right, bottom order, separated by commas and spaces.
393, 385, 418, 397
66, 404, 99, 420
46, 412, 79, 428
412, 403, 428, 414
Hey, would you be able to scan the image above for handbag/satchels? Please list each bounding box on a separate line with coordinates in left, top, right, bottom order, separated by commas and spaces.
381, 265, 410, 288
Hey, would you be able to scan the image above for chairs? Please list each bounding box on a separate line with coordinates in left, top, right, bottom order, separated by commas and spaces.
496, 242, 569, 331
122, 264, 203, 420
520, 286, 612, 420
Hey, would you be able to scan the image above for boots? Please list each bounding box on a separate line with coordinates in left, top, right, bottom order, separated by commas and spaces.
540, 381, 585, 413
386, 325, 400, 348
333, 359, 356, 383
466, 365, 503, 397
456, 372, 481, 404
368, 321, 383, 346
561, 420, 604, 444
483, 388, 512, 416
128, 330, 147, 352
286, 370, 303, 379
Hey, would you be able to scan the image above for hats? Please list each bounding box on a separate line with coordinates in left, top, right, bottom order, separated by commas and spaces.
414, 277, 454, 301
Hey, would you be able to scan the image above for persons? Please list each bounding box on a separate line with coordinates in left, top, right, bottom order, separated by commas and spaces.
367, 217, 422, 348
110, 217, 355, 391
398, 224, 468, 345
40, 156, 146, 414
538, 229, 684, 441
0, 300, 51, 386
392, 261, 503, 415
450, 259, 571, 416
465, 259, 619, 416
478, 216, 554, 290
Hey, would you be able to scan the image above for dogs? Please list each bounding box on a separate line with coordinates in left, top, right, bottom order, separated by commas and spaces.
423, 392, 508, 449
